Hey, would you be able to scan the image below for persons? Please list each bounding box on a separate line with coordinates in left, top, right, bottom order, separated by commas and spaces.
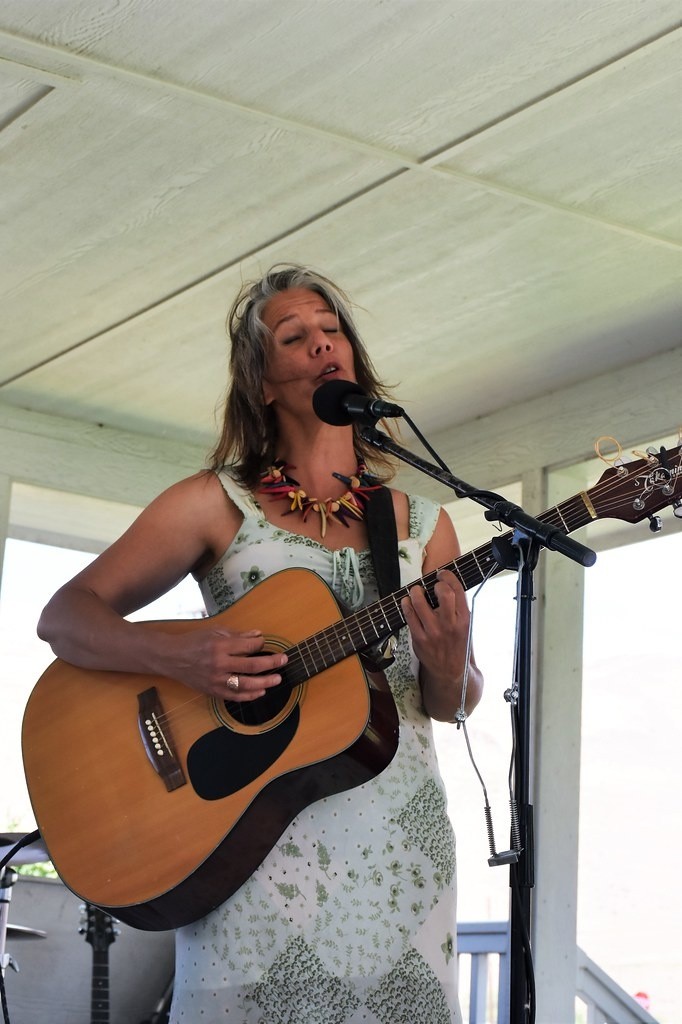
37, 263, 482, 1024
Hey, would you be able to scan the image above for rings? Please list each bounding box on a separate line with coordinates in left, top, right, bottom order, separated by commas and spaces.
227, 674, 239, 692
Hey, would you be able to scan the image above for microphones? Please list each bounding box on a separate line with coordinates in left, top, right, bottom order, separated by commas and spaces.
311, 379, 404, 427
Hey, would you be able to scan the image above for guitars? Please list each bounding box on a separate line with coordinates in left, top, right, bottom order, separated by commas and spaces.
21, 434, 682, 933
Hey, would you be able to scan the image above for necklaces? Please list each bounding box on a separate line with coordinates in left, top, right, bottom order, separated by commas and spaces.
260, 456, 382, 537
